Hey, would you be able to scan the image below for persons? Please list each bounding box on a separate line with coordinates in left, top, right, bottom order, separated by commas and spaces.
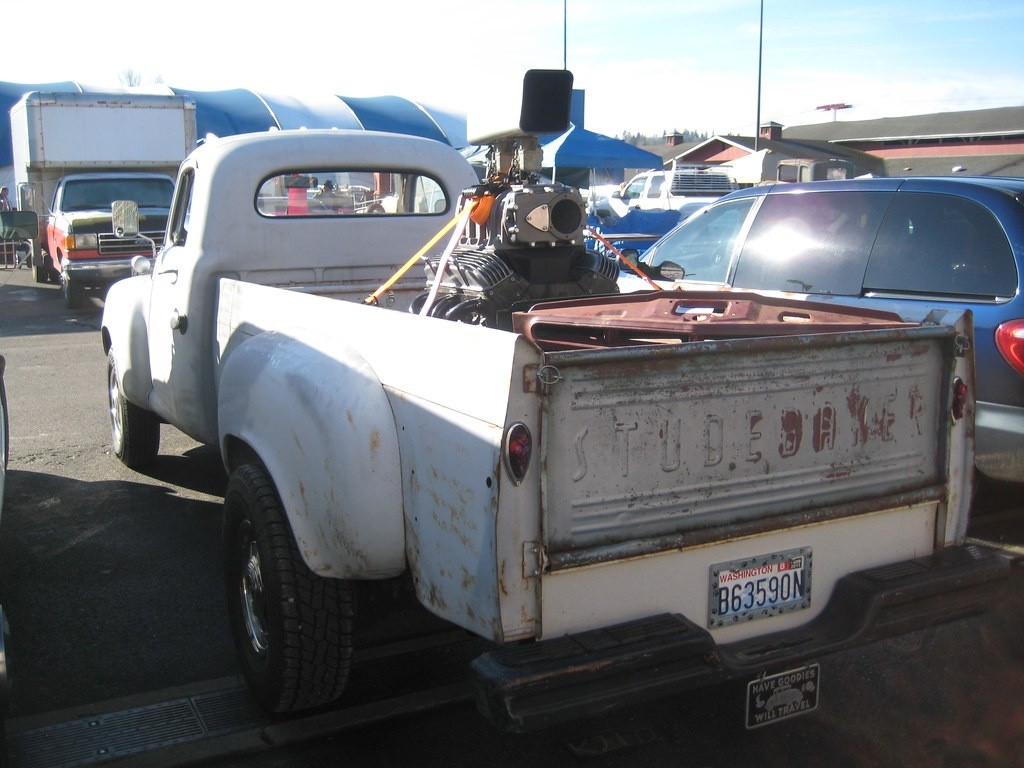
282, 169, 387, 216
0, 186, 12, 215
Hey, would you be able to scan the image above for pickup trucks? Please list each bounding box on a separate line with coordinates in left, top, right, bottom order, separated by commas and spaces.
101, 128, 1010, 733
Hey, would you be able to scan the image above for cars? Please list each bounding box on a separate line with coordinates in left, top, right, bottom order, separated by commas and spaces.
613, 169, 1024, 482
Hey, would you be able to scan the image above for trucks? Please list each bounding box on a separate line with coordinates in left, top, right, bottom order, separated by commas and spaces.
10, 86, 196, 308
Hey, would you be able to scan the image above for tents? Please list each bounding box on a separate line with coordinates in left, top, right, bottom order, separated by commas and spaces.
0, 80, 469, 228
525, 129, 670, 217
